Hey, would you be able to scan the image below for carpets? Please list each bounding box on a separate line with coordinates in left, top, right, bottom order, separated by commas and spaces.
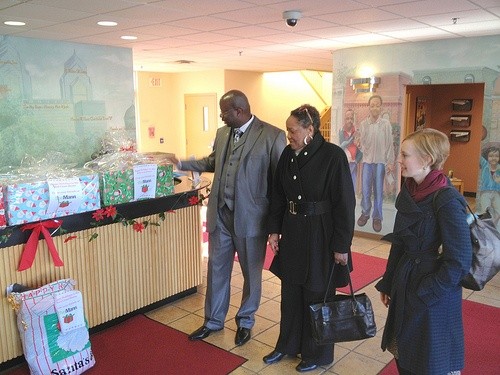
234, 244, 390, 294
376, 299, 500, 375
7, 313, 249, 375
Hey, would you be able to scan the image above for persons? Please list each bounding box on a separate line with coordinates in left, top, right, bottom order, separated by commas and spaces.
374, 128, 473, 375
339, 108, 361, 201
477, 148, 500, 236
262, 104, 357, 372
357, 95, 393, 232
166, 89, 287, 346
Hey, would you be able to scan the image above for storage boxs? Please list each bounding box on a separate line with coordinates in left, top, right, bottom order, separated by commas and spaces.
2, 172, 100, 226
97, 163, 175, 206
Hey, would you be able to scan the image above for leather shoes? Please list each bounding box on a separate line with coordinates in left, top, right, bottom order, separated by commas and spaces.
235, 327, 251, 345
263, 349, 285, 364
296, 360, 317, 371
189, 325, 225, 341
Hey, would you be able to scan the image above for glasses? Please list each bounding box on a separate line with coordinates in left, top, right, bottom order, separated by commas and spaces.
299, 106, 314, 124
220, 110, 231, 118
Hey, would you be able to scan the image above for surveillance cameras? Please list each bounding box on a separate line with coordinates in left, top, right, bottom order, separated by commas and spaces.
282, 10, 302, 26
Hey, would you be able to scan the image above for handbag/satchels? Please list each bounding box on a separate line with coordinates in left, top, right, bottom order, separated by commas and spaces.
308, 260, 377, 342
431, 186, 500, 291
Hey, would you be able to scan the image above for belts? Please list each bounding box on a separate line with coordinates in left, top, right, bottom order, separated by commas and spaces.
285, 200, 331, 217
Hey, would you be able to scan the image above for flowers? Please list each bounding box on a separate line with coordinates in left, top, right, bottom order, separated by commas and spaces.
0, 190, 210, 243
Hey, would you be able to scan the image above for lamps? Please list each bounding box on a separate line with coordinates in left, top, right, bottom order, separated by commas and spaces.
350, 77, 380, 93
